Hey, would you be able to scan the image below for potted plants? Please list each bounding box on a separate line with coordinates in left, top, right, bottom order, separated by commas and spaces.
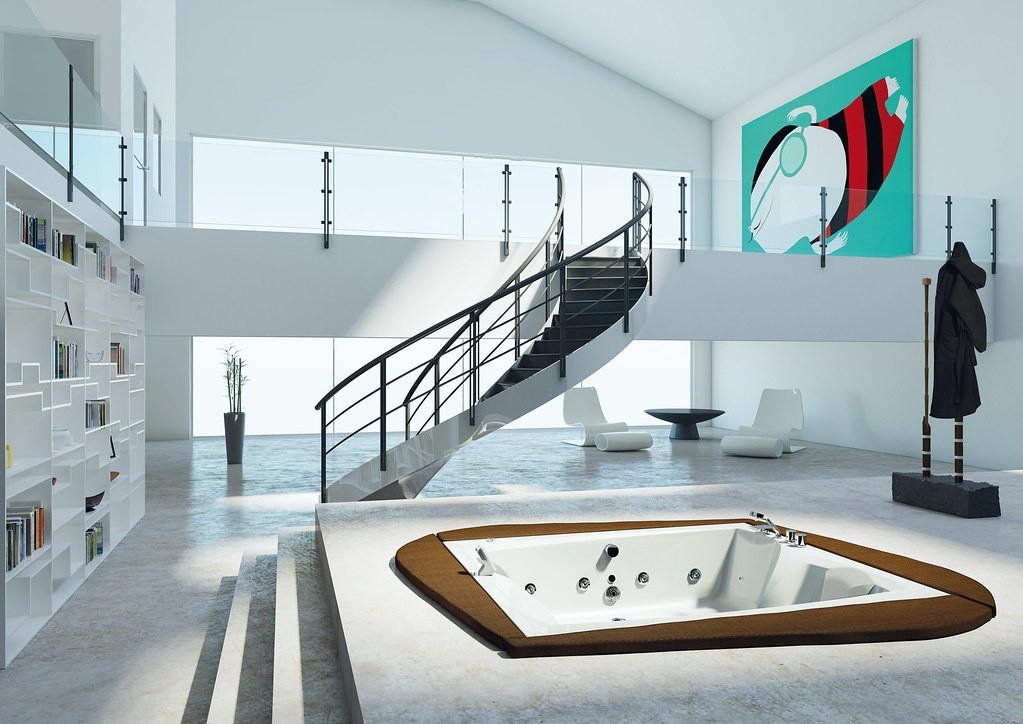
217, 341, 251, 464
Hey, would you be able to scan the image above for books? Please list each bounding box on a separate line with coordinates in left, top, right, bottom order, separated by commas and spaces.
1, 213, 144, 575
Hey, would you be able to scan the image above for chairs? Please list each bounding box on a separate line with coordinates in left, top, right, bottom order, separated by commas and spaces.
737, 388, 808, 454
562, 386, 628, 447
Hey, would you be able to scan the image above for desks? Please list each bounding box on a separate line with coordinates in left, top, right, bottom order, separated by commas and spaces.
645, 408, 726, 439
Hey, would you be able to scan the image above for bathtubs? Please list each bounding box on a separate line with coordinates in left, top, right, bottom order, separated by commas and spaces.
394, 508, 1001, 664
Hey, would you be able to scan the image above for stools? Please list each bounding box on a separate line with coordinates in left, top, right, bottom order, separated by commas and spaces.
596, 432, 654, 451
720, 436, 785, 458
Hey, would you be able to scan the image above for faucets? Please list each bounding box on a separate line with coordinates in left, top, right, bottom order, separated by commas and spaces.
747, 511, 785, 539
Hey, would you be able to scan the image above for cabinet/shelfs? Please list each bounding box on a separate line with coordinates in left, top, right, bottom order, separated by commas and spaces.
2, 163, 143, 661
